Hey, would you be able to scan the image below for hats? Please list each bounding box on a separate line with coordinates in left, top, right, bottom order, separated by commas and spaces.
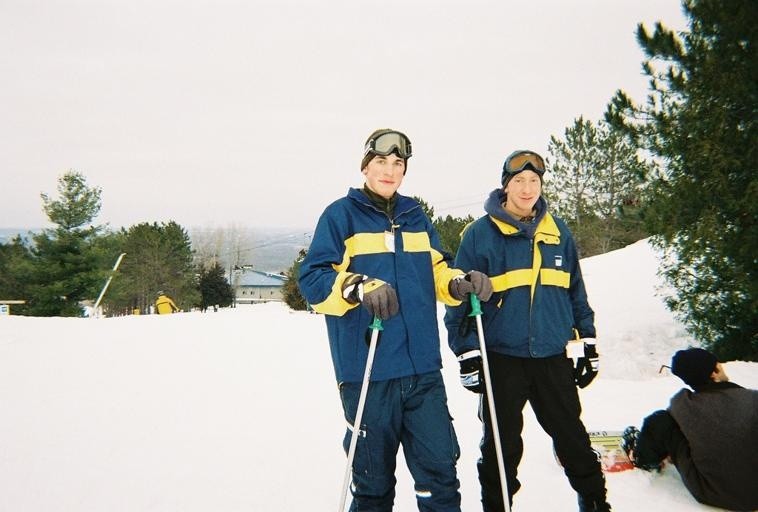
671, 348, 717, 390
501, 149, 544, 192
361, 128, 408, 176
157, 291, 164, 296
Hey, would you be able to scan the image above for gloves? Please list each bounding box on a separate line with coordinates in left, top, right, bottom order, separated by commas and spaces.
456, 348, 487, 395
575, 337, 600, 389
449, 270, 493, 303
342, 273, 399, 321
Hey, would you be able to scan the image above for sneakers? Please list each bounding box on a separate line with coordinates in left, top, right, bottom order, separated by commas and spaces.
622, 426, 659, 471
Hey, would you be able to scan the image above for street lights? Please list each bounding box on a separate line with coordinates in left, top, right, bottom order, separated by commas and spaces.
233, 264, 254, 307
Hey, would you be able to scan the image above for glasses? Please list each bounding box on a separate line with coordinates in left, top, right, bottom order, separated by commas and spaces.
500, 151, 546, 184
361, 130, 413, 160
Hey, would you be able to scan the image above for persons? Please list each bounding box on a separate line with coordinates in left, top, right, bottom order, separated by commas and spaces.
623, 348, 758, 512
298, 127, 492, 510
442, 148, 615, 510
153, 290, 180, 314
